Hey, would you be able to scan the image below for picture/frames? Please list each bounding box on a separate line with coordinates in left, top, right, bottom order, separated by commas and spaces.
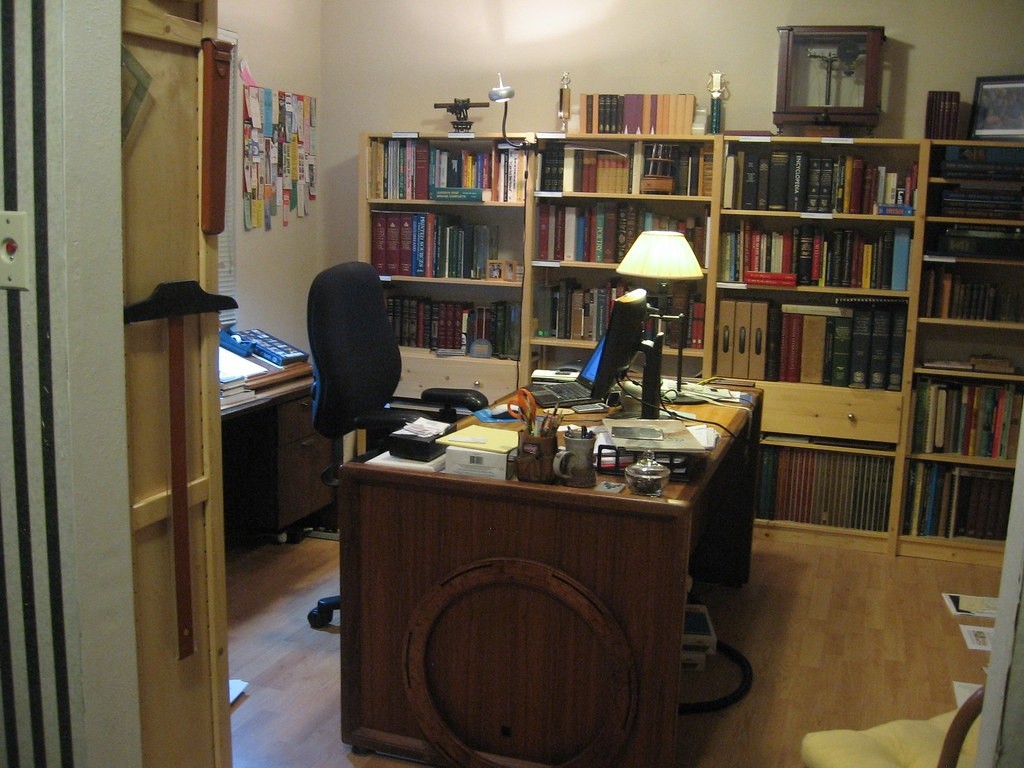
970, 76, 1024, 139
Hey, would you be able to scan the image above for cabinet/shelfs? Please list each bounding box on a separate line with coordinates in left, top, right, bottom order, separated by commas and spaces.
356, 131, 1023, 565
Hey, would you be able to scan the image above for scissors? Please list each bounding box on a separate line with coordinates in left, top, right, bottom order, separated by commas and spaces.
506, 388, 536, 435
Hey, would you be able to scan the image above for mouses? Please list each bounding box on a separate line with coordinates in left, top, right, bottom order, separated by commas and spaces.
491, 404, 519, 419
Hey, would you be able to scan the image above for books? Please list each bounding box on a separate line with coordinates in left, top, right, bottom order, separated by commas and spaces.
372, 138, 1024, 541
219, 371, 255, 406
579, 93, 697, 135
925, 91, 960, 140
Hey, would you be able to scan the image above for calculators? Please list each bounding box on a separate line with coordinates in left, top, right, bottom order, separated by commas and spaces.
572, 402, 610, 414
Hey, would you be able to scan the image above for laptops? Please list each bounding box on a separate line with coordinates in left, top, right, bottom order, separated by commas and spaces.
524, 334, 606, 408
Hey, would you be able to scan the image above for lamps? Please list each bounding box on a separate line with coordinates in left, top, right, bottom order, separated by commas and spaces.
487, 76, 537, 152
620, 230, 708, 406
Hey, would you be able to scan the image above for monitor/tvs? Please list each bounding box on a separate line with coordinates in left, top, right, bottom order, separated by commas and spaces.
590, 288, 672, 421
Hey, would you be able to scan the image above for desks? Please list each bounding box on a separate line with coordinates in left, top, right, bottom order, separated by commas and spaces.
220, 380, 317, 546
338, 385, 764, 768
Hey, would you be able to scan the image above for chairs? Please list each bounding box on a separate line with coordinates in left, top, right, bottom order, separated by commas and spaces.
306, 260, 486, 627
801, 685, 986, 763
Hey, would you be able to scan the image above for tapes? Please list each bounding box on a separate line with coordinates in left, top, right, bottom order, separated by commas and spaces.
230, 335, 242, 344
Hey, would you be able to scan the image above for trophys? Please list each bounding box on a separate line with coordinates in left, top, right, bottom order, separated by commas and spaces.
558, 71, 572, 132
706, 70, 726, 135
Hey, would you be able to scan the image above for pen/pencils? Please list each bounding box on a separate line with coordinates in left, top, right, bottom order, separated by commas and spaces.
517, 398, 564, 438
564, 424, 595, 439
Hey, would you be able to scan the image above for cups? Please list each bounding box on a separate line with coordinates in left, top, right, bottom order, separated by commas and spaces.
553, 431, 596, 488
516, 430, 558, 483
